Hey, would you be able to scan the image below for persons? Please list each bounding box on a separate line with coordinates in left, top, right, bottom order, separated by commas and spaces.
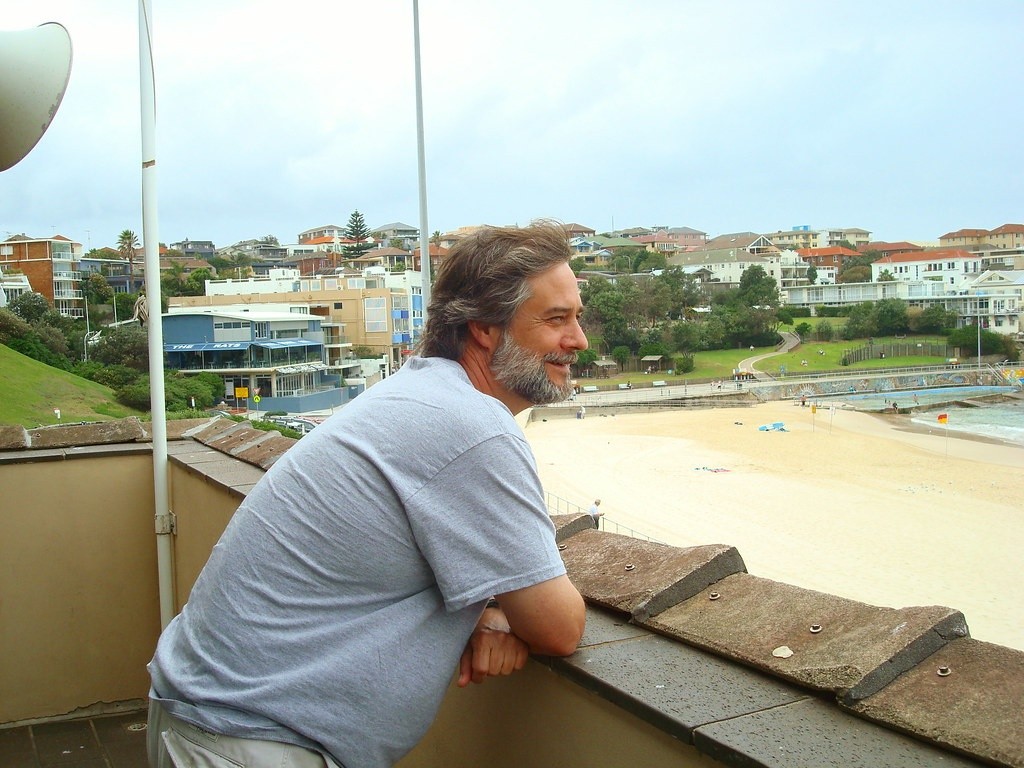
709, 379, 723, 391
579, 405, 585, 419
801, 394, 806, 409
647, 363, 658, 375
627, 381, 631, 390
800, 359, 808, 367
912, 393, 918, 403
589, 498, 606, 531
850, 386, 853, 391
892, 400, 898, 415
145, 226, 589, 768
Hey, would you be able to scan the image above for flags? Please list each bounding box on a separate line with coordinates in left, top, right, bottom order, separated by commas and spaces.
937, 413, 948, 425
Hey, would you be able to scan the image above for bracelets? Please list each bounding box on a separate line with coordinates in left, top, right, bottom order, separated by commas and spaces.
486, 598, 499, 608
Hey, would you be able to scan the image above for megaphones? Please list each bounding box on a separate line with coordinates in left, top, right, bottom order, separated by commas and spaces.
0, 22, 73, 170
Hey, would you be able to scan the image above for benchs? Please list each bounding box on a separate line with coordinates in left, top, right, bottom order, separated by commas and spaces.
652, 380, 667, 387
618, 384, 632, 390
582, 386, 600, 393
